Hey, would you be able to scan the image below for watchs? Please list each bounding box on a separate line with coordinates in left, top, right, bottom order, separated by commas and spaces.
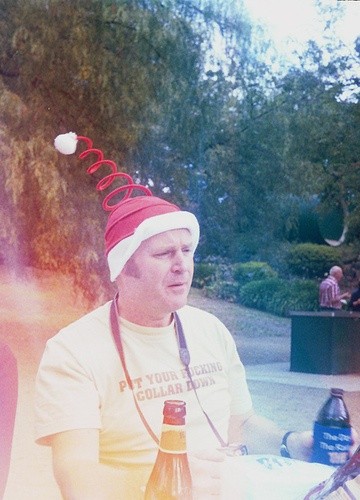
280, 431, 293, 458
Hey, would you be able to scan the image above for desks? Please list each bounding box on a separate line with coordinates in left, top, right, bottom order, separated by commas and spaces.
291, 310, 360, 374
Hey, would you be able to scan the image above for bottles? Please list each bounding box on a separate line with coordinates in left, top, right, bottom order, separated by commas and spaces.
310, 387, 353, 469
143, 400, 194, 500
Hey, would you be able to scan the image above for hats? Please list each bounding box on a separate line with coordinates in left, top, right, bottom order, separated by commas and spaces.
53, 131, 201, 281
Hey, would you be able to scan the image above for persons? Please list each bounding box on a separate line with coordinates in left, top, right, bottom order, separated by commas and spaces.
33, 195, 357, 500
348, 282, 360, 312
318, 265, 351, 311
0, 343, 18, 500
